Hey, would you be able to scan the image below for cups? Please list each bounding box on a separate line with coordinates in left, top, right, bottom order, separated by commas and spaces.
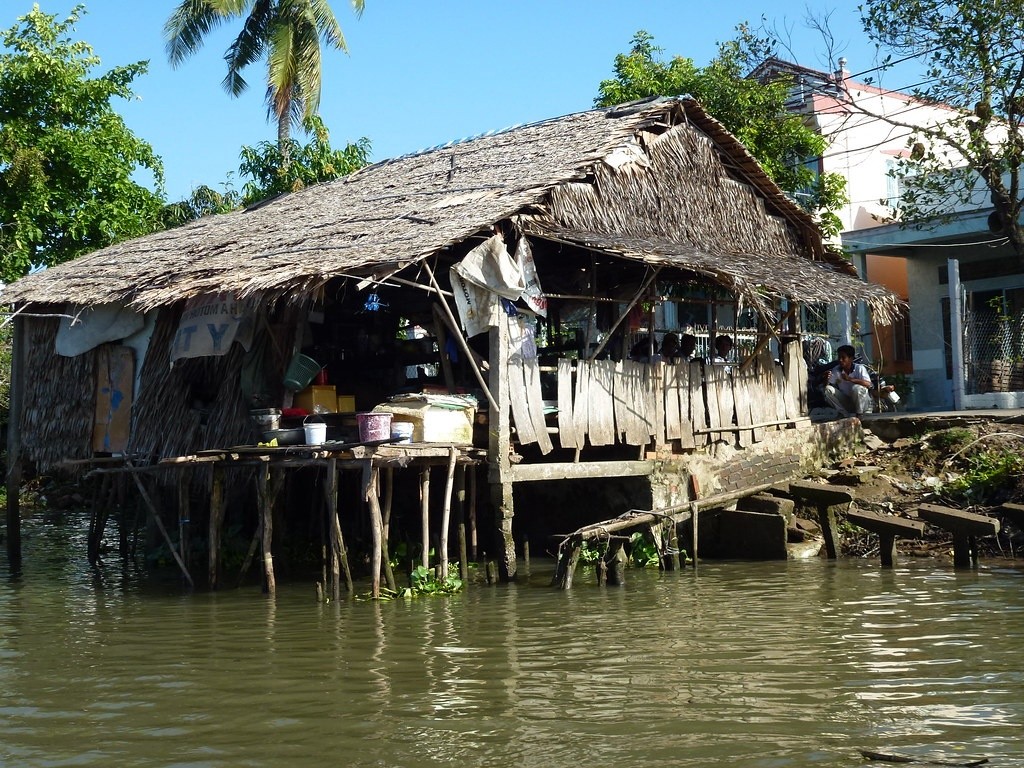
316, 370, 328, 385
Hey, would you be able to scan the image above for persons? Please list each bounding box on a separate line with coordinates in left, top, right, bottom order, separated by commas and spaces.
629, 332, 696, 365
823, 345, 873, 416
715, 335, 733, 360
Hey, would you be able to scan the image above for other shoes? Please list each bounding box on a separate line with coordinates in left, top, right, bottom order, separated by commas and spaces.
834, 412, 849, 420
856, 413, 864, 420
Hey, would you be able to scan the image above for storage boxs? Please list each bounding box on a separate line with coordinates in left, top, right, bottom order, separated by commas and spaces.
374, 402, 473, 443
294, 385, 355, 413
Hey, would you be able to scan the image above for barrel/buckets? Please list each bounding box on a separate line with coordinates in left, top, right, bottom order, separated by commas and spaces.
390, 421, 415, 445
248, 408, 283, 444
303, 413, 327, 446
355, 412, 394, 443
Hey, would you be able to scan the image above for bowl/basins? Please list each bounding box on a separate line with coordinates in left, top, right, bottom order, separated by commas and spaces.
262, 428, 305, 447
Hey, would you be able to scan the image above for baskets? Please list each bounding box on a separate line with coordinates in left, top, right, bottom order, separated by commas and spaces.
283, 344, 328, 392
